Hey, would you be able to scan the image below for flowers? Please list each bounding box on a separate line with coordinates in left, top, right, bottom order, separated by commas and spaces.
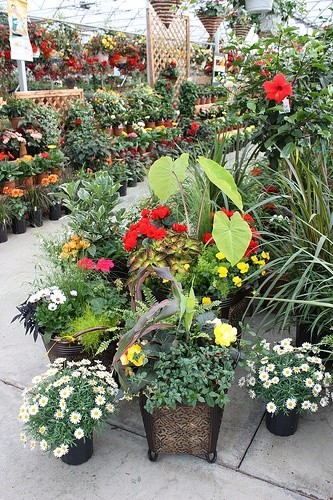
0, 0, 333, 459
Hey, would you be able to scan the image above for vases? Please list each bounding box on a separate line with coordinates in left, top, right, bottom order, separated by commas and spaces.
295, 313, 333, 376
193, 288, 250, 343
60, 428, 95, 466
243, 0, 273, 14
139, 390, 225, 464
265, 407, 299, 437
38, 329, 119, 366
